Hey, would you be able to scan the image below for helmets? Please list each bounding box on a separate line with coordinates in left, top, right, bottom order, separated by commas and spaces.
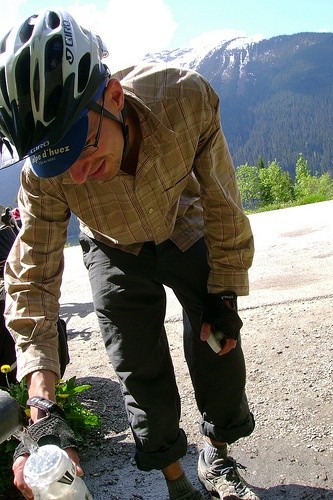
0, 9, 109, 178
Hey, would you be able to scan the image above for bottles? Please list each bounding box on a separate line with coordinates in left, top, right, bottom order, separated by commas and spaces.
24, 445, 93, 500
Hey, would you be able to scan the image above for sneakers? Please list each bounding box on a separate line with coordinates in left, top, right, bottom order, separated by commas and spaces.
197, 448, 261, 500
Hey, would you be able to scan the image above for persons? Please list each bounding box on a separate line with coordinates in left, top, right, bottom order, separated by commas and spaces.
1, 12, 256, 499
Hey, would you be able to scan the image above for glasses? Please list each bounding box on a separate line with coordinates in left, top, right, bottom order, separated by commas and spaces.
77, 87, 105, 159
